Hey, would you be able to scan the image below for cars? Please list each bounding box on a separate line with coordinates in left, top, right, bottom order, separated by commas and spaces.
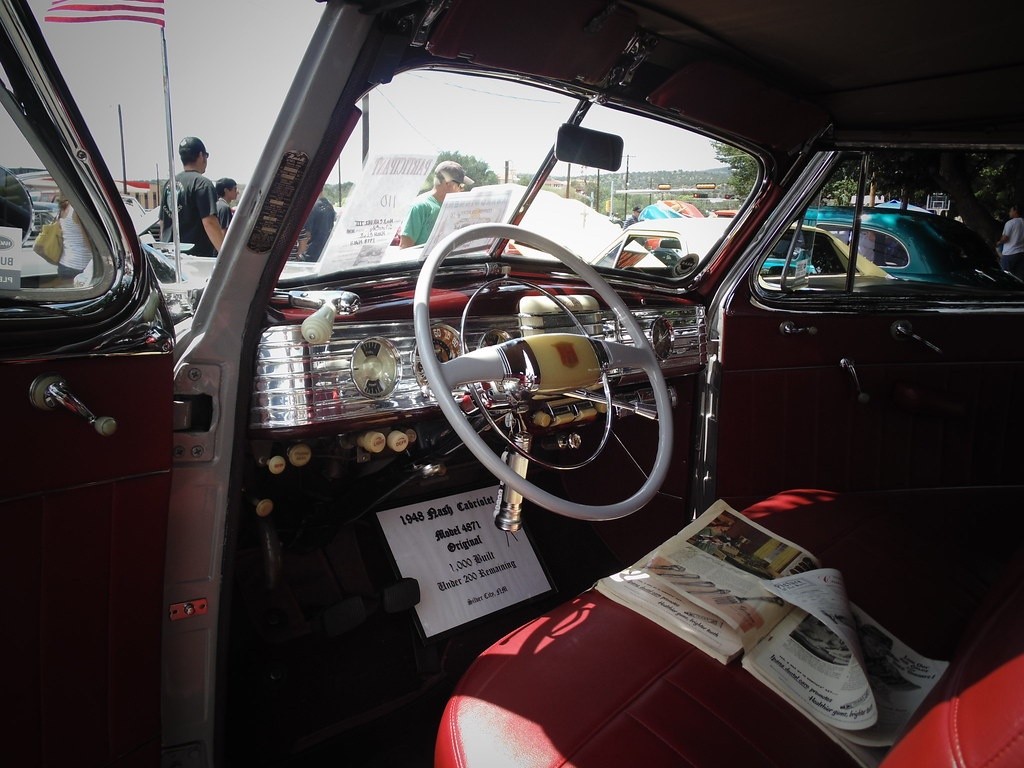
800, 208, 1024, 292
588, 217, 925, 294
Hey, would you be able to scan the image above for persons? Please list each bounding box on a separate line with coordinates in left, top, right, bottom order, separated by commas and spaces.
399, 161, 474, 249
858, 622, 898, 692
994, 204, 1024, 281
295, 188, 335, 263
941, 210, 954, 220
216, 178, 239, 236
706, 210, 717, 218
158, 137, 224, 257
623, 206, 642, 229
57, 188, 92, 287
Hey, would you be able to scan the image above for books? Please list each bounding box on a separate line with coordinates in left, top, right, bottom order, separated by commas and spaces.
595, 499, 949, 768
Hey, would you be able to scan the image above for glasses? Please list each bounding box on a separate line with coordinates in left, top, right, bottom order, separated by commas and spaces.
636, 211, 642, 213
222, 186, 239, 193
197, 150, 208, 158
452, 180, 465, 189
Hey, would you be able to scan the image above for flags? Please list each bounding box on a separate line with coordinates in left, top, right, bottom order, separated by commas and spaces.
44, 0, 165, 26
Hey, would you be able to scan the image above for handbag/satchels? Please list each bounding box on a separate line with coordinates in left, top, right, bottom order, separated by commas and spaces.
33, 210, 62, 266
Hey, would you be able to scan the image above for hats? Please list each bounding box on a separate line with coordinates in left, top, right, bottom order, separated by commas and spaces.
435, 161, 475, 185
179, 136, 206, 151
632, 206, 640, 211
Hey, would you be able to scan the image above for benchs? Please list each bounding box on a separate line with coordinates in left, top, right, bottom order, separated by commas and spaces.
432, 487, 1024, 768
